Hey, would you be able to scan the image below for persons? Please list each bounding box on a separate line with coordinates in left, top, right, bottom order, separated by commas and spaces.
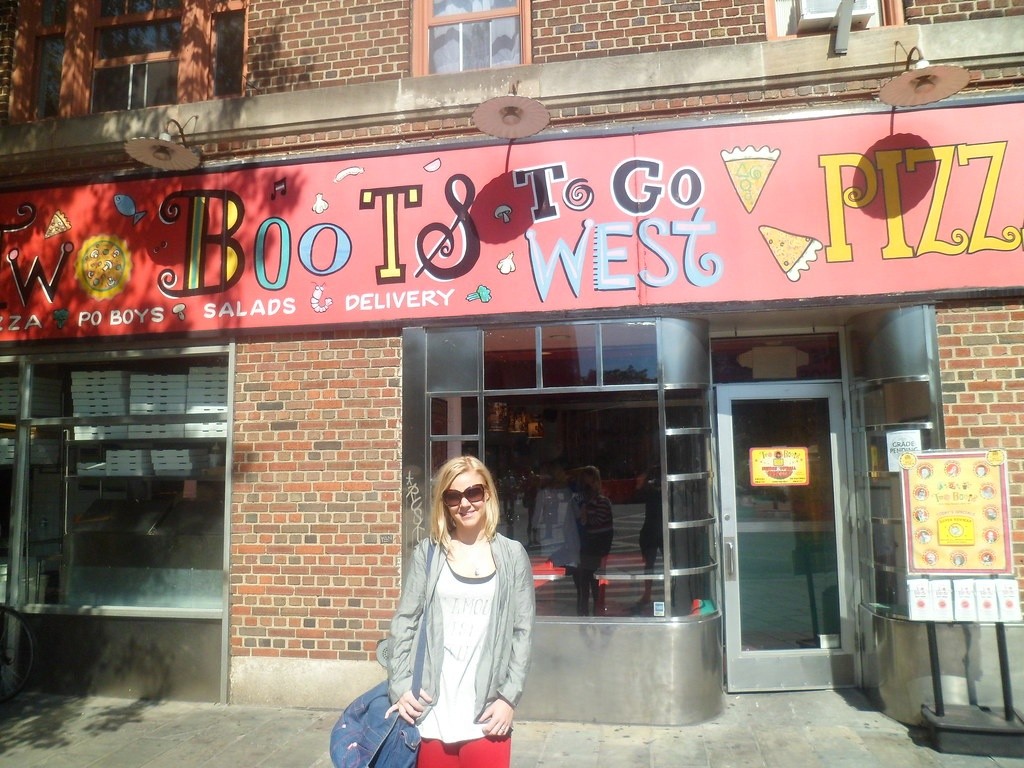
382, 455, 537, 768
653, 430, 691, 473
499, 460, 696, 617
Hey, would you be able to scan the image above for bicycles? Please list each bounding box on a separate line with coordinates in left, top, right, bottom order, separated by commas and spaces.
0, 603, 38, 704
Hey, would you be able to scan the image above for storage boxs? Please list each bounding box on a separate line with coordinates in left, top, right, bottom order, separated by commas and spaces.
0, 366, 229, 604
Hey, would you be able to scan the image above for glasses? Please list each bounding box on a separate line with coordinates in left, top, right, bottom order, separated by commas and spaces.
442, 484, 486, 507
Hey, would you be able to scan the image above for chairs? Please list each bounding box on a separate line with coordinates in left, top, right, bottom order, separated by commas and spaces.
596, 553, 647, 616
529, 555, 554, 615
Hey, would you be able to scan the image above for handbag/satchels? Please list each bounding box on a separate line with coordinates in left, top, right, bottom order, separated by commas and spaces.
547, 546, 580, 576
330, 681, 421, 767
522, 495, 532, 508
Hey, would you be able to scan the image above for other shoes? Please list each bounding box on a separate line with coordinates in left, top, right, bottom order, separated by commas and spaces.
638, 595, 651, 606
528, 541, 540, 547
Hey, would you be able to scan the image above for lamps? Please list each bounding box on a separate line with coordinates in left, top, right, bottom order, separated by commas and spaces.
124, 119, 199, 171
878, 45, 968, 108
471, 86, 551, 140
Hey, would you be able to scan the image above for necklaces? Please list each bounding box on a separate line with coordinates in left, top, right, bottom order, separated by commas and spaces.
473, 567, 480, 577
